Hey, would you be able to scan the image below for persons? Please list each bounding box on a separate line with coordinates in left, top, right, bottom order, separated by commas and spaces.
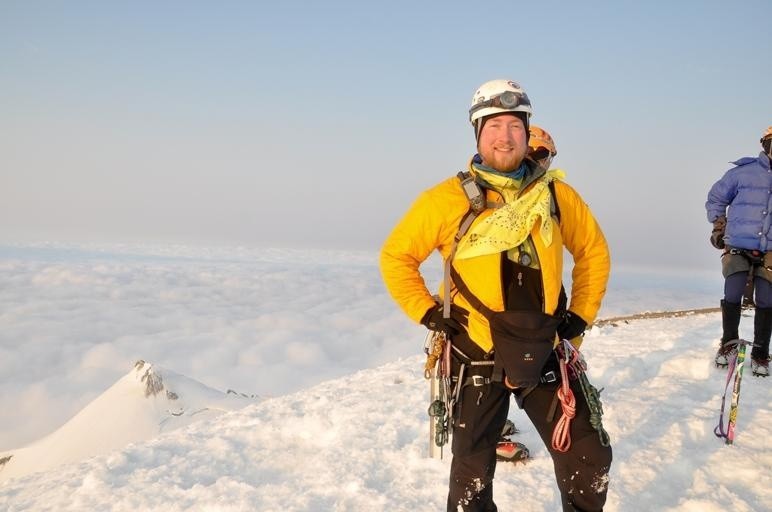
380, 79, 613, 512
452, 126, 557, 462
705, 126, 772, 377
742, 281, 757, 310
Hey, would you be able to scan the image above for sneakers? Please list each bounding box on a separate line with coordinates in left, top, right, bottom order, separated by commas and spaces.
716, 346, 739, 367
751, 358, 771, 377
495, 439, 530, 462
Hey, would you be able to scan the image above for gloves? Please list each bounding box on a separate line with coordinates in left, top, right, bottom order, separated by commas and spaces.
552, 310, 587, 342
421, 301, 471, 338
711, 216, 726, 250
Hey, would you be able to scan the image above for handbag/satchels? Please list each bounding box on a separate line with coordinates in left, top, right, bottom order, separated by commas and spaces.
488, 310, 557, 388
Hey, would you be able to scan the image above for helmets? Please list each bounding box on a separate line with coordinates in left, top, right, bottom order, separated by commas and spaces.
760, 125, 771, 143
469, 79, 533, 126
